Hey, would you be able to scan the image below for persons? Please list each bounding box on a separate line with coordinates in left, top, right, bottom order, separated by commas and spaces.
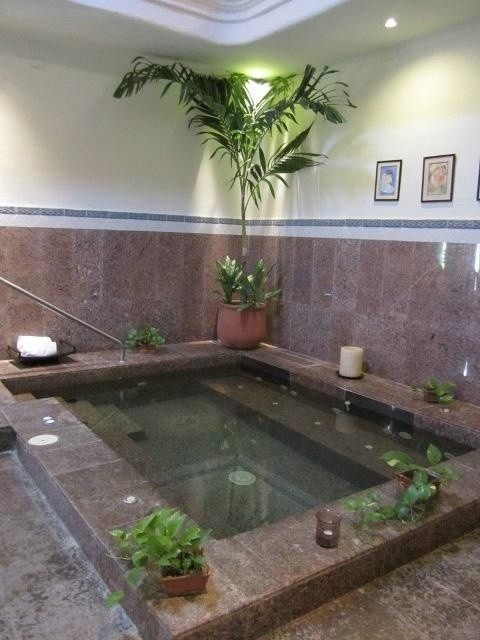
428, 162, 449, 196
378, 165, 397, 197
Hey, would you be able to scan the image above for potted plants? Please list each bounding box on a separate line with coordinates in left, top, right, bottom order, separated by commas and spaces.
343, 442, 463, 525
95, 502, 217, 610
408, 375, 459, 406
108, 54, 360, 353
121, 324, 167, 354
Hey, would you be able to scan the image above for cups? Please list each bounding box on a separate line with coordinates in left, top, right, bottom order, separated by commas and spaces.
334, 411, 360, 434
315, 508, 342, 548
339, 346, 363, 377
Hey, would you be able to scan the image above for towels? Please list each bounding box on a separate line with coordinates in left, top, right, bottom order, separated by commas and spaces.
16, 334, 57, 357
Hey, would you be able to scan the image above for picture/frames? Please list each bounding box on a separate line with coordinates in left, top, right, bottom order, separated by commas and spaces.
476, 159, 480, 203
371, 158, 402, 201
419, 154, 455, 203
